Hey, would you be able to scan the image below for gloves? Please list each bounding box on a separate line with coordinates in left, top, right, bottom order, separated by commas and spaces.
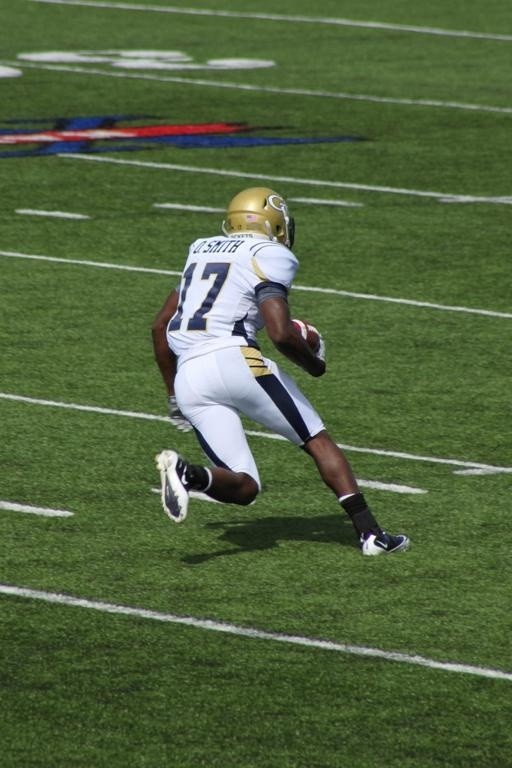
310, 331, 326, 369
166, 395, 194, 433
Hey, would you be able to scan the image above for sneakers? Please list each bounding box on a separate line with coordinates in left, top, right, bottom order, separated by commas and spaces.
359, 530, 410, 557
155, 449, 191, 524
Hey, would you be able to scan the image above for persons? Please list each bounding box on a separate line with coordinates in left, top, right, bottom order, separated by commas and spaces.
149, 184, 414, 559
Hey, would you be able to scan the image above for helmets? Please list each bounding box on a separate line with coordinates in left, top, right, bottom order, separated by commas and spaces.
221, 186, 294, 249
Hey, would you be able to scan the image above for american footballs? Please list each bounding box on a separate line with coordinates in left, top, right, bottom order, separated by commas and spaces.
290, 319, 321, 353
